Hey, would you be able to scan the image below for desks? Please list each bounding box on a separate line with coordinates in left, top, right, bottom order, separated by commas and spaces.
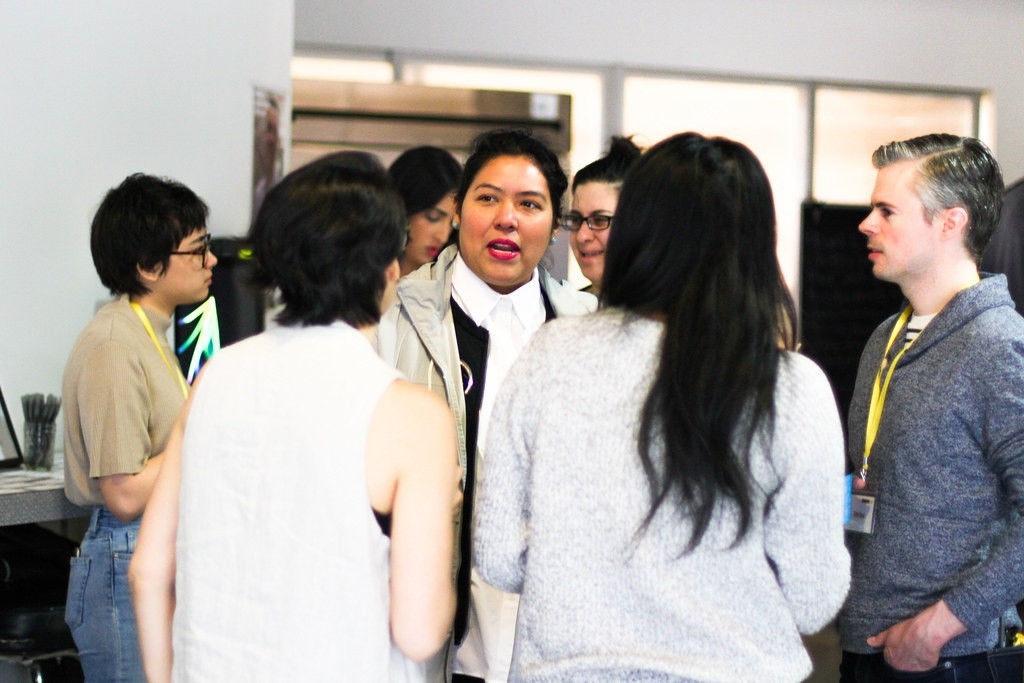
0, 453, 93, 524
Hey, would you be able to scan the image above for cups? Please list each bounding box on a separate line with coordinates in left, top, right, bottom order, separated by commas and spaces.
19, 420, 56, 472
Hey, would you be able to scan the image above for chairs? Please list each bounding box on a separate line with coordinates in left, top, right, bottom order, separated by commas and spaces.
0, 523, 79, 683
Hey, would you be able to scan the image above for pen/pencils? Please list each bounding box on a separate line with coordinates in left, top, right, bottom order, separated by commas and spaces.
21, 394, 62, 470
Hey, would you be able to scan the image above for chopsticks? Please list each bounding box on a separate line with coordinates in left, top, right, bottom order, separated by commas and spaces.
21, 393, 62, 422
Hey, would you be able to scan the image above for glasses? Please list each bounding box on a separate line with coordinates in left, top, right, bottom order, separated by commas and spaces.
170, 234, 212, 268
562, 214, 617, 232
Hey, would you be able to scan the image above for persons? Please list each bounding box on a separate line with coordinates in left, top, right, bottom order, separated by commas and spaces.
370, 130, 644, 683
63, 173, 218, 683
128, 150, 463, 683
839, 134, 1024, 683
472, 132, 852, 683
